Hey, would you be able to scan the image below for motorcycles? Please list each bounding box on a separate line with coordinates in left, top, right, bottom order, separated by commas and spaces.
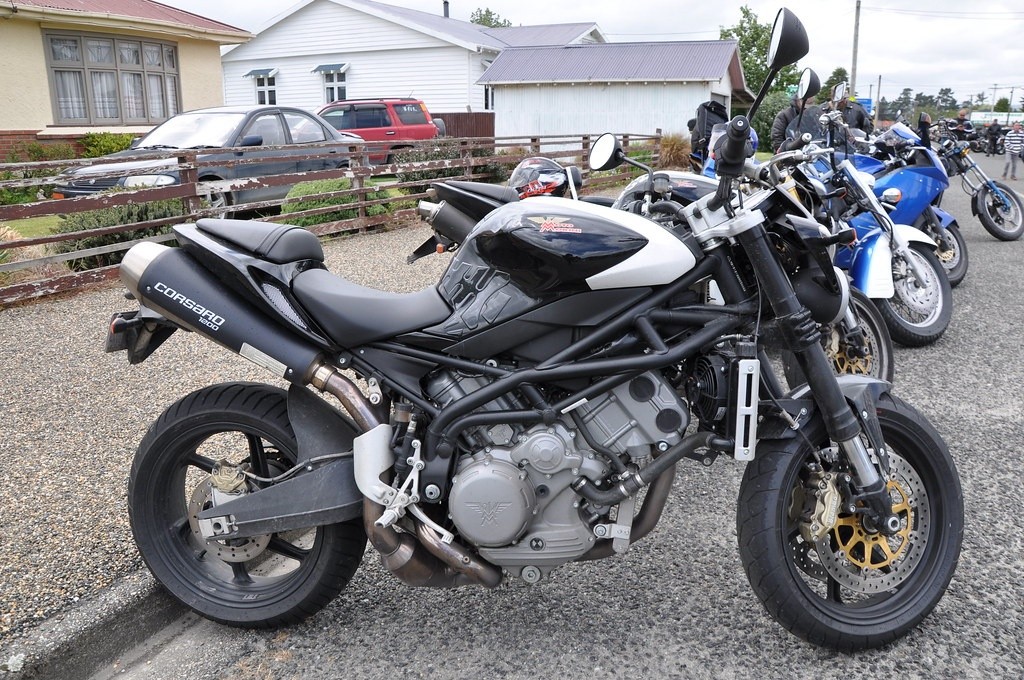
103, 1, 1024, 654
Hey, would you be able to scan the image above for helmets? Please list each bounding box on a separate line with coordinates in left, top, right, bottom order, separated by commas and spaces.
508, 157, 568, 197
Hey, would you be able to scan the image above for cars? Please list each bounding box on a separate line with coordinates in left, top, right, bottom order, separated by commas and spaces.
55, 103, 372, 221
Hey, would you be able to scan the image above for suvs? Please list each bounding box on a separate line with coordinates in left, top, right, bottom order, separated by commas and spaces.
293, 96, 445, 166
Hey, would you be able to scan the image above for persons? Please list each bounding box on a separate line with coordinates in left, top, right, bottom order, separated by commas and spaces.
985, 119, 1002, 157
771, 91, 874, 155
917, 112, 931, 149
895, 110, 904, 123
1001, 122, 1024, 180
948, 110, 973, 142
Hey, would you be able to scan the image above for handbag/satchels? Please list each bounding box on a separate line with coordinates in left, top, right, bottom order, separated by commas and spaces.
691, 101, 728, 159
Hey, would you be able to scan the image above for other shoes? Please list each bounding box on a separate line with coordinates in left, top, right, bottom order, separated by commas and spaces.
986, 155, 989, 157
1002, 175, 1006, 180
1011, 176, 1016, 180
